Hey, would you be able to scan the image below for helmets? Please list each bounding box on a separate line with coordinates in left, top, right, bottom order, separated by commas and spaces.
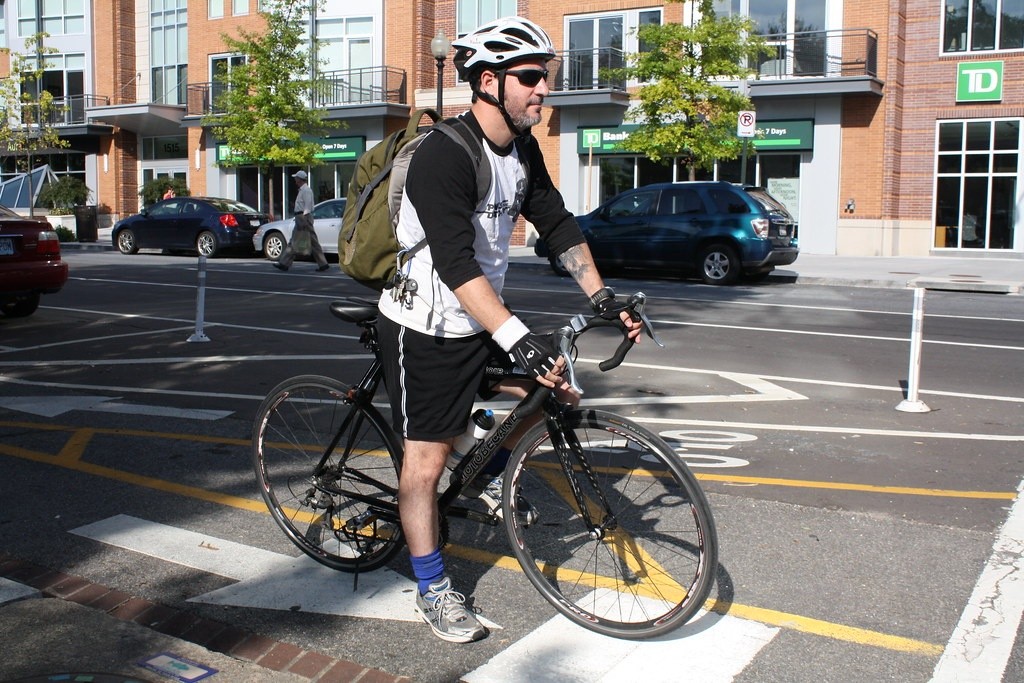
451, 16, 557, 82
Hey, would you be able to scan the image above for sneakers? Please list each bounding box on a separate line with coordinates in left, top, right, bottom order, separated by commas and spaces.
449, 468, 540, 526
415, 577, 485, 643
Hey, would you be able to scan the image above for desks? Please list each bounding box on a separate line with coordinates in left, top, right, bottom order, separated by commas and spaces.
935, 226, 965, 248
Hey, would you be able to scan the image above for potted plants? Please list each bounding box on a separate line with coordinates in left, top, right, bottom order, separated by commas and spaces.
45, 206, 76, 238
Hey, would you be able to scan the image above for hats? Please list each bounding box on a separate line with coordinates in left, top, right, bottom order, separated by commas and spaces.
291, 170, 308, 180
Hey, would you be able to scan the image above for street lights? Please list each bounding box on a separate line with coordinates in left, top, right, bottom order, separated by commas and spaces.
430, 29, 450, 118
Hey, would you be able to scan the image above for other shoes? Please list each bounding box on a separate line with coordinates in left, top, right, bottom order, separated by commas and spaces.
314, 264, 330, 272
273, 263, 289, 271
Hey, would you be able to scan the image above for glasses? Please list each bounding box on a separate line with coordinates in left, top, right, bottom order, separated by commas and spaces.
495, 69, 549, 88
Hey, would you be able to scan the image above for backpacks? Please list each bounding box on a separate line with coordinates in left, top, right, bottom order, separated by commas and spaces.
337, 108, 491, 292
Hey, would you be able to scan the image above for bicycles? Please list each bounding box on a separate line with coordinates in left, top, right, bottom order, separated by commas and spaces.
251, 290, 718, 641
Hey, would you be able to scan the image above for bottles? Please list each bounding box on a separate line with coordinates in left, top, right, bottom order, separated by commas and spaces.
445, 409, 496, 473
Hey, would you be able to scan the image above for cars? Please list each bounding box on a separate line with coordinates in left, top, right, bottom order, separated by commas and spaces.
253, 198, 348, 261
112, 197, 274, 258
0, 205, 69, 316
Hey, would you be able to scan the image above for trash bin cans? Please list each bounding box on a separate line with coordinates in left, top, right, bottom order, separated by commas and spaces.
74, 205, 98, 242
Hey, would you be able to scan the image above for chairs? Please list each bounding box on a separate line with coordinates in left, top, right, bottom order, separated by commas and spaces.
184, 204, 194, 212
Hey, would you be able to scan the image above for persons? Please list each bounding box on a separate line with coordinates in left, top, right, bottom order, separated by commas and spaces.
163, 186, 176, 200
376, 16, 642, 643
273, 171, 330, 272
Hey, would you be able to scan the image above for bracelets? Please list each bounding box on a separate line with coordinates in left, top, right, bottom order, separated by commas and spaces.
588, 286, 615, 308
492, 315, 530, 352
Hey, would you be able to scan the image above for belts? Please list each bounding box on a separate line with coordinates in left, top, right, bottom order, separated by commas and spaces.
295, 211, 303, 215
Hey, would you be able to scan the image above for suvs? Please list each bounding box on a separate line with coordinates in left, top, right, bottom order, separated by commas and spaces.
535, 180, 799, 285
